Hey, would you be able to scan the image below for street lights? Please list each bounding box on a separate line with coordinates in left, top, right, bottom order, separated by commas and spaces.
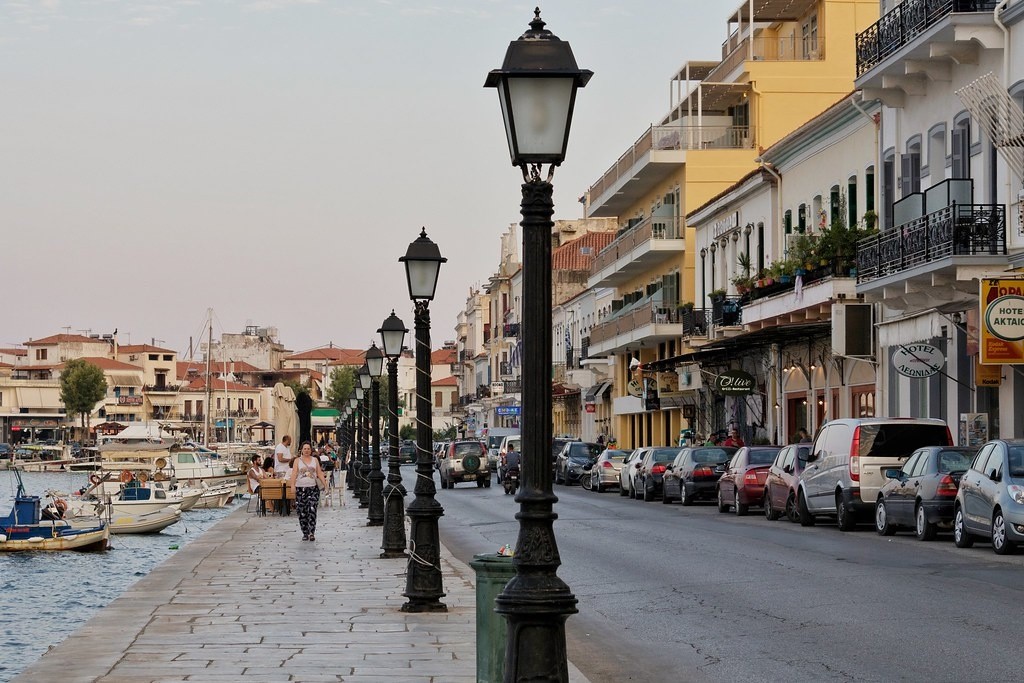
481, 6, 594, 683
394, 226, 449, 612
377, 309, 418, 564
334, 341, 385, 527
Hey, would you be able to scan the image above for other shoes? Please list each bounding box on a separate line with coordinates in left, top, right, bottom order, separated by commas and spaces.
302, 534, 316, 541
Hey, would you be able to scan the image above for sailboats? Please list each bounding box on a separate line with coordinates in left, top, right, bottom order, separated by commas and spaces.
100, 305, 251, 499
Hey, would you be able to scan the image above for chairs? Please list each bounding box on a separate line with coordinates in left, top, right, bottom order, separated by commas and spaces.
695, 455, 712, 462
719, 453, 727, 461
326, 470, 347, 506
258, 478, 285, 517
245, 474, 278, 513
286, 480, 299, 516
655, 454, 668, 460
318, 471, 332, 507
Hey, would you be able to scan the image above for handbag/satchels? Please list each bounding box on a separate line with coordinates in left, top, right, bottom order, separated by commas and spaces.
316, 477, 325, 491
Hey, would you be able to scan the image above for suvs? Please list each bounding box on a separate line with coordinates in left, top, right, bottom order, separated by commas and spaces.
438, 437, 492, 490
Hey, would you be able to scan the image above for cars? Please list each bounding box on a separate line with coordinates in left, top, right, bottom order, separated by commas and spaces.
70, 443, 82, 457
13, 444, 37, 459
361, 431, 741, 506
952, 439, 1024, 555
714, 445, 786, 516
0, 443, 14, 458
762, 442, 814, 523
39, 440, 60, 459
873, 445, 979, 541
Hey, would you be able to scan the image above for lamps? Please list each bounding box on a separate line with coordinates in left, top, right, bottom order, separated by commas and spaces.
952, 312, 966, 329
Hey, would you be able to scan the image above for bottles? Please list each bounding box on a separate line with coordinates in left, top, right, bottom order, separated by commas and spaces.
495, 544, 513, 555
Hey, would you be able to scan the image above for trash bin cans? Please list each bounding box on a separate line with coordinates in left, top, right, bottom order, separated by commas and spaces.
469, 553, 518, 683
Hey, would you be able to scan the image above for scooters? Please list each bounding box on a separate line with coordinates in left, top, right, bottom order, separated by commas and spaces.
498, 457, 520, 496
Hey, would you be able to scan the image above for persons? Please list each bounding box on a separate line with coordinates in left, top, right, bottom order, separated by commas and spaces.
291, 441, 327, 541
501, 444, 521, 486
799, 428, 813, 443
681, 428, 744, 448
381, 443, 388, 456
596, 434, 620, 450
170, 475, 177, 491
247, 435, 352, 512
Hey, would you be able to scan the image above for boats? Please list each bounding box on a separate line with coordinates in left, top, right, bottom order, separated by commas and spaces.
44, 475, 237, 536
62, 447, 101, 473
0, 455, 112, 556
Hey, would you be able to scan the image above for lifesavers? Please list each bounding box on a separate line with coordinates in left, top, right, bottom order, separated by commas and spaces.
90, 475, 100, 484
241, 462, 250, 473
55, 499, 67, 511
122, 470, 131, 482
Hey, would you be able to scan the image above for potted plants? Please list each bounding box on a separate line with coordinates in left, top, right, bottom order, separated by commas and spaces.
675, 287, 728, 314
729, 209, 877, 294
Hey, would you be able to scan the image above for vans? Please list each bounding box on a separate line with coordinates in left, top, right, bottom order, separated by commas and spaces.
797, 417, 955, 533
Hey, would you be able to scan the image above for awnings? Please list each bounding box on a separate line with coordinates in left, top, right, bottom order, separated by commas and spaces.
585, 382, 612, 404
637, 321, 832, 373
